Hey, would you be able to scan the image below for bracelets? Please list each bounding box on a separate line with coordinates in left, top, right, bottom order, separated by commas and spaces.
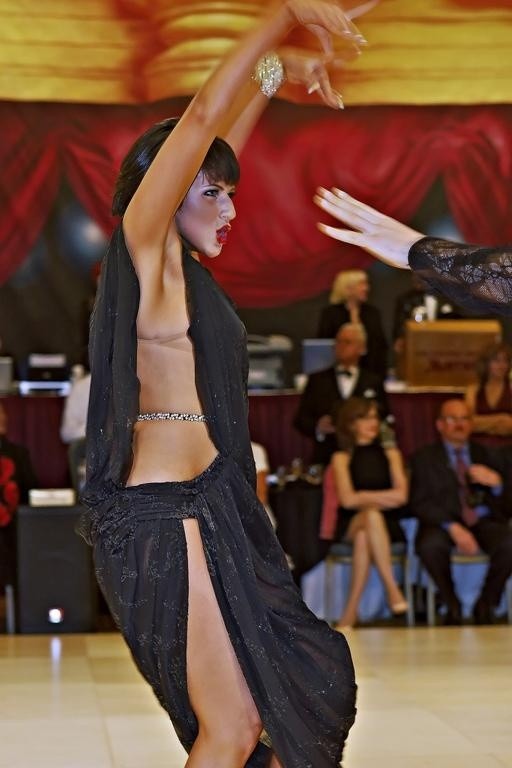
255, 51, 288, 101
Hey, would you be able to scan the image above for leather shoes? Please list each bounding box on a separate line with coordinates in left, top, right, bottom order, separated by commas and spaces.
441, 599, 504, 625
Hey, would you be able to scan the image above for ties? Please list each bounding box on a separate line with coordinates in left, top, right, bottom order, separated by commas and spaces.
453, 447, 477, 525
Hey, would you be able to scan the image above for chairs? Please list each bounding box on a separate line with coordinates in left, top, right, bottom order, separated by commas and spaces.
19, 508, 512, 634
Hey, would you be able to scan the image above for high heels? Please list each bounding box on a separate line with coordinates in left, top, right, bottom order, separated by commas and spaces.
387, 591, 408, 615
335, 615, 357, 633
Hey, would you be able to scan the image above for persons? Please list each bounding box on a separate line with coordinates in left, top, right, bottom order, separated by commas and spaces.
307, 178, 511, 305
77, 0, 381, 768
294, 322, 386, 465
317, 270, 390, 381
330, 398, 412, 632
408, 399, 511, 624
